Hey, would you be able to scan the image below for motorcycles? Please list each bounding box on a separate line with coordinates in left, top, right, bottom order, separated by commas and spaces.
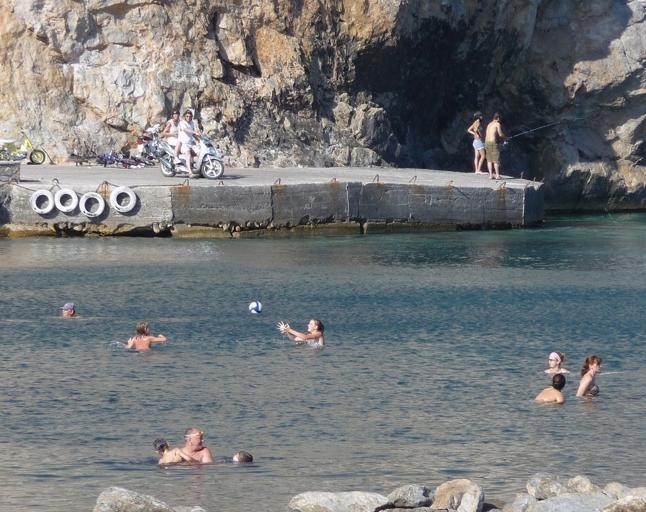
0, 122, 45, 165
155, 133, 224, 180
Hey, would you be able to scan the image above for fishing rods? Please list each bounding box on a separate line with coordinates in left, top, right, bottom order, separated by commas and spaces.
506, 118, 581, 139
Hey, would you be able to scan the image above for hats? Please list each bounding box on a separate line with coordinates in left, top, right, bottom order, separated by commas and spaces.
553, 374, 565, 387
60, 303, 76, 312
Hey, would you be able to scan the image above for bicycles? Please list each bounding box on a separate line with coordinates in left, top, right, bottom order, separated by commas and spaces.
71, 143, 161, 169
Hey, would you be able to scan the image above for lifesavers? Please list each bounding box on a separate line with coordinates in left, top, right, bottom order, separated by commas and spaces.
30, 190, 54, 215
110, 186, 136, 212
55, 188, 78, 212
79, 192, 104, 218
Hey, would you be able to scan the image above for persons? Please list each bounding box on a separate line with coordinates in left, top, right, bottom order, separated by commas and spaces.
232, 450, 253, 463
161, 110, 182, 164
276, 317, 325, 347
575, 355, 605, 397
543, 351, 571, 374
180, 430, 215, 464
122, 322, 168, 352
534, 374, 566, 404
152, 438, 193, 468
177, 109, 203, 179
485, 111, 507, 179
467, 111, 488, 175
58, 302, 81, 320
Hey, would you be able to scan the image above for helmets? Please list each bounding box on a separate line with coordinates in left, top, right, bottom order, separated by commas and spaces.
473, 111, 482, 119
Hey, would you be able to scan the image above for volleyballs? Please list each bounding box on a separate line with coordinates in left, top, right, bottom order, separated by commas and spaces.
249, 301, 262, 314
